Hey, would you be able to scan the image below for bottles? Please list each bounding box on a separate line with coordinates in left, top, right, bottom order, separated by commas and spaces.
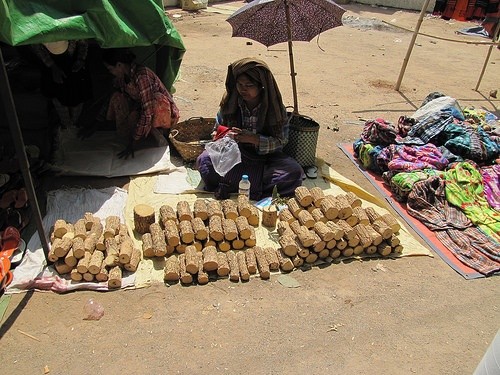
238, 175, 251, 205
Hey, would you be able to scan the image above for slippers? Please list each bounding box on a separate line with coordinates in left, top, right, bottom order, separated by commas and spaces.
0, 140, 38, 295
299, 166, 318, 180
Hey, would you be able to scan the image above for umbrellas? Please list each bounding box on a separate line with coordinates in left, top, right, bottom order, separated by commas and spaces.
225, 0, 347, 128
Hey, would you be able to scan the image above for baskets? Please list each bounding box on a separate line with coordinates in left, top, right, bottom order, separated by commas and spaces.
282, 105, 321, 167
169, 116, 217, 163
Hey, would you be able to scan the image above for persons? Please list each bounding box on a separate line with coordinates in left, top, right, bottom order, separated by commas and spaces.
197, 57, 303, 198
101, 52, 180, 161
33, 39, 90, 126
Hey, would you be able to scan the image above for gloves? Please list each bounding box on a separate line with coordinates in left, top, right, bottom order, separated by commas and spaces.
76, 122, 98, 142
116, 143, 135, 161
